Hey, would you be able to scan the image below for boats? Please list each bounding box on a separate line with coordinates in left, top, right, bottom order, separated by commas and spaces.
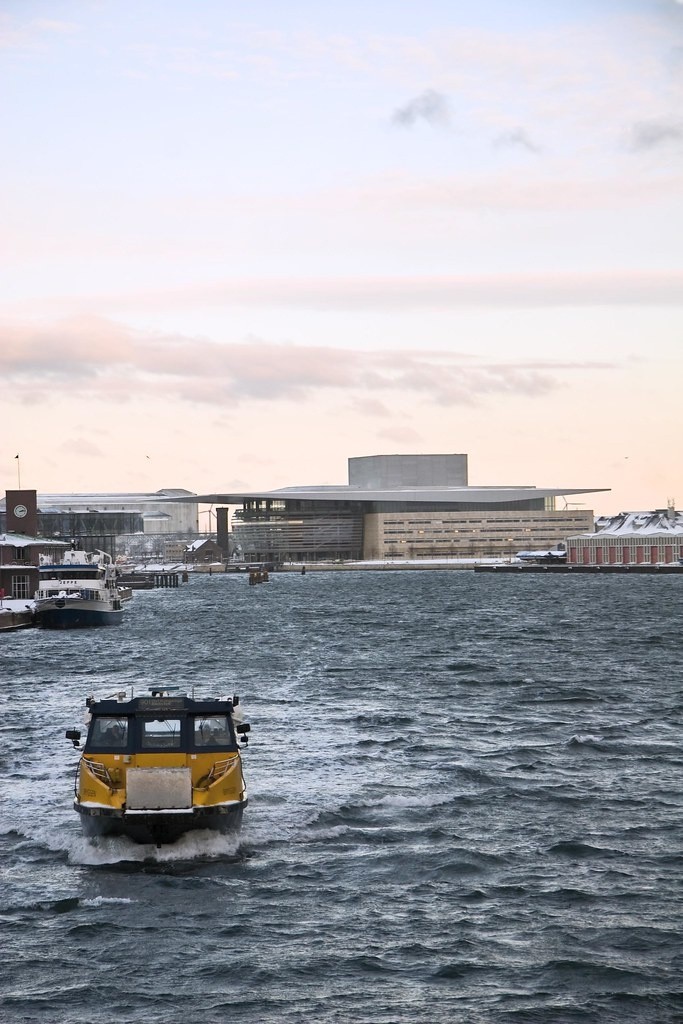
32, 540, 124, 630
65, 684, 252, 845
